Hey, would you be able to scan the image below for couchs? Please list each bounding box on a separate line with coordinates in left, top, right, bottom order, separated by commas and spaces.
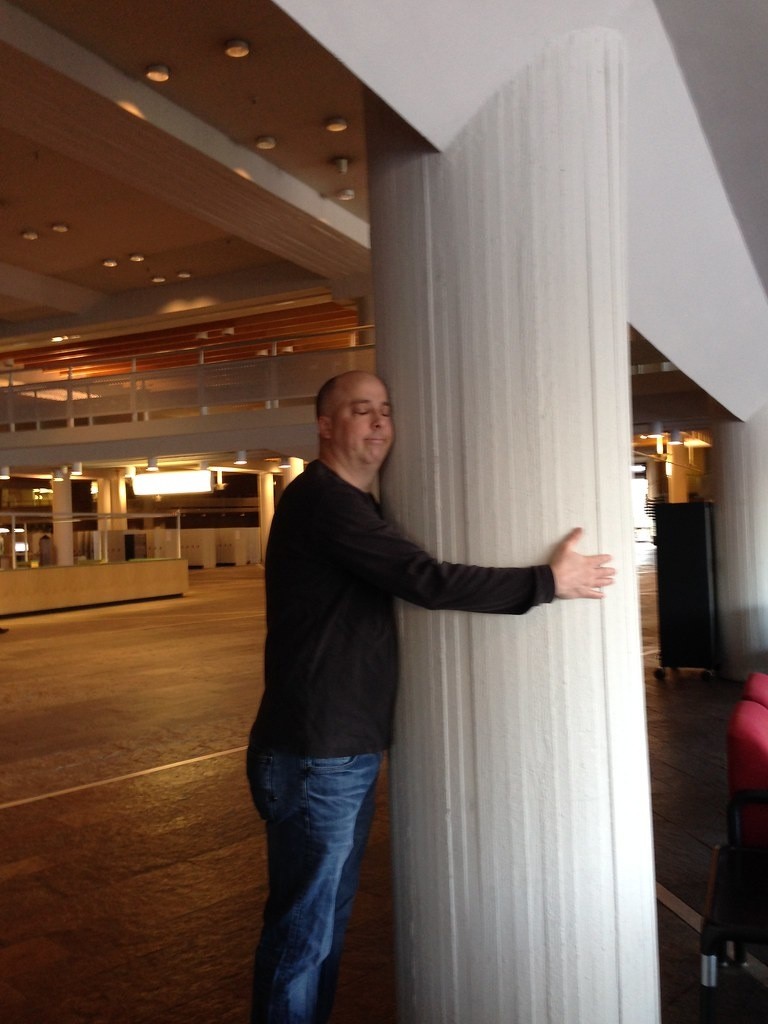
697, 672, 768, 988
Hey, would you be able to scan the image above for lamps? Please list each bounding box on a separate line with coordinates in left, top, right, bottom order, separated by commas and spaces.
0, 467, 10, 480
235, 451, 247, 465
280, 456, 292, 468
148, 457, 158, 471
53, 461, 82, 481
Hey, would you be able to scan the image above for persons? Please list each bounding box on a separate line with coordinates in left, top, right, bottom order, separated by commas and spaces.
245, 371, 617, 1024
